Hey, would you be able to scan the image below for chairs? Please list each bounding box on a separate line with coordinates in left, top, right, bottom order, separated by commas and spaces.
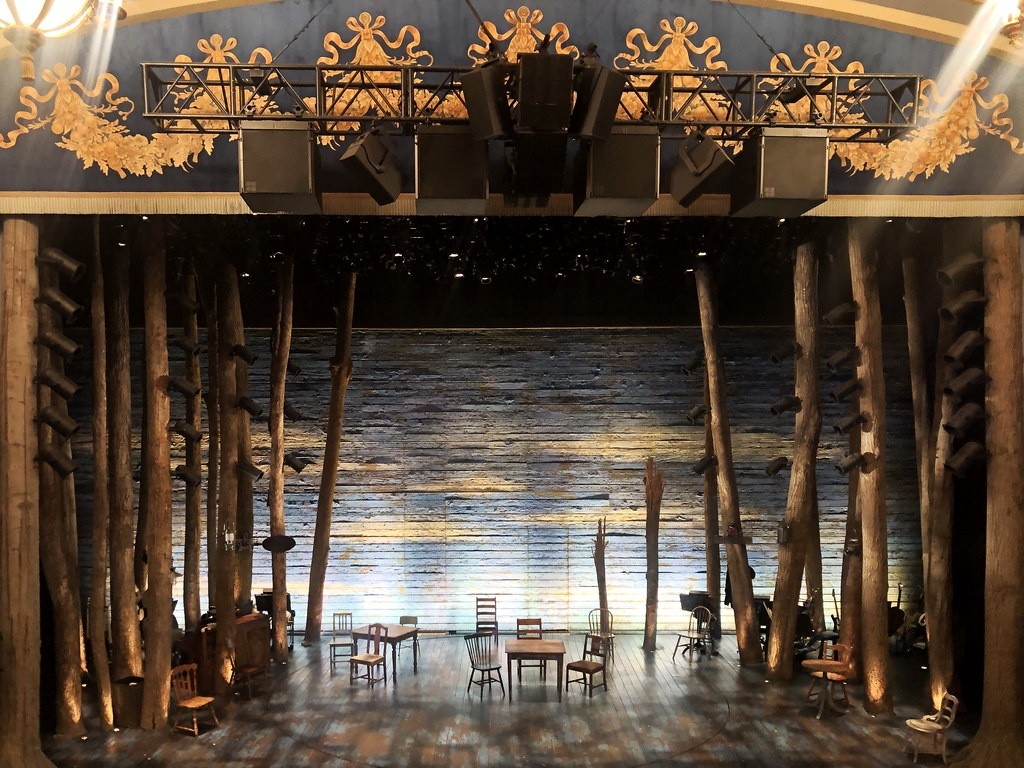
589, 608, 616, 663
464, 631, 505, 700
254, 588, 297, 647
566, 633, 608, 697
220, 637, 266, 701
820, 644, 857, 706
831, 614, 841, 633
518, 618, 546, 682
475, 597, 500, 646
903, 695, 960, 765
328, 613, 355, 670
169, 663, 218, 736
348, 622, 390, 689
399, 617, 421, 655
672, 606, 710, 663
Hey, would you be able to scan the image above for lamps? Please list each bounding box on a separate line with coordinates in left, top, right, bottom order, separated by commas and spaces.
172, 420, 203, 447
172, 335, 203, 357
233, 343, 260, 365
241, 398, 263, 418
284, 402, 304, 423
289, 361, 303, 378
685, 250, 1000, 555
283, 454, 307, 473
167, 378, 201, 403
31, 248, 77, 473
237, 460, 264, 482
173, 463, 204, 487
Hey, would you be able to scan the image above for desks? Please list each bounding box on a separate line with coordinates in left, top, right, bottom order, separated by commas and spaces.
352, 624, 419, 682
801, 658, 852, 719
505, 640, 567, 699
816, 631, 839, 659
